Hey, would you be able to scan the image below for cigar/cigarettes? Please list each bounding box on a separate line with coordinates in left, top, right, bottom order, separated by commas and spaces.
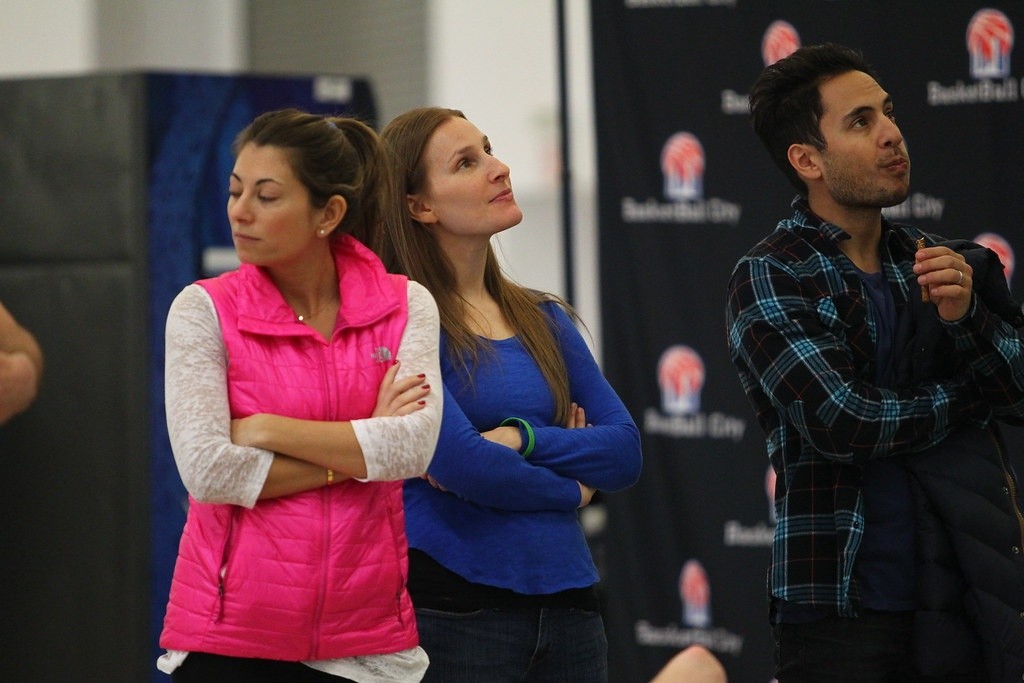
916, 239, 929, 302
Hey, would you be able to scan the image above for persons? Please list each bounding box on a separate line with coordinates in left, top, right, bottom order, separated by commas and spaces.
725, 48, 1024, 683
378, 107, 643, 682
156, 113, 442, 683
0, 305, 41, 421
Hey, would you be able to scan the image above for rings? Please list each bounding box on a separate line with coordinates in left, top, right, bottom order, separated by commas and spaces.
957, 270, 963, 284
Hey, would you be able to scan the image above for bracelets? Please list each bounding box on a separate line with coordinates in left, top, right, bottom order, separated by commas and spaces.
328, 469, 334, 486
500, 417, 534, 458
